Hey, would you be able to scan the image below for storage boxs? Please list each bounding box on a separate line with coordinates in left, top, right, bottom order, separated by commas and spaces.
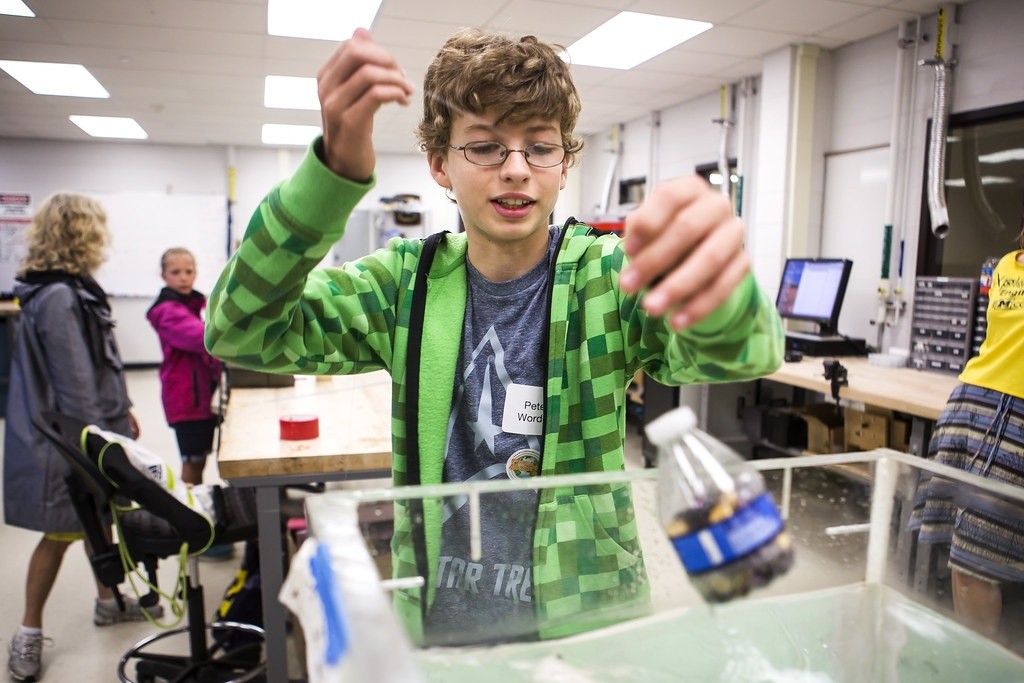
786, 402, 905, 456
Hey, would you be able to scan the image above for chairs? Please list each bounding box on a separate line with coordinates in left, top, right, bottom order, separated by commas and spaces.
32, 411, 267, 683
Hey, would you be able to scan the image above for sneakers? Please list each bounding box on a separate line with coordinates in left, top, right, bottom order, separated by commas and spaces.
94, 593, 165, 626
8, 628, 54, 681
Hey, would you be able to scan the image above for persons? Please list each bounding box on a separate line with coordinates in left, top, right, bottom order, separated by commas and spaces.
144, 248, 226, 512
910, 246, 1024, 641
200, 29, 789, 660
0, 191, 166, 683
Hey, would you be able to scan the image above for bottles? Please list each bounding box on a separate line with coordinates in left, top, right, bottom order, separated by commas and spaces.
644, 405, 794, 604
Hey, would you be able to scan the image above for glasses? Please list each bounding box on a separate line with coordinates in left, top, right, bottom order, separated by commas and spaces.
445, 141, 567, 168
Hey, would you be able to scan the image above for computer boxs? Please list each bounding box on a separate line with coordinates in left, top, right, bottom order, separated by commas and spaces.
786, 331, 866, 357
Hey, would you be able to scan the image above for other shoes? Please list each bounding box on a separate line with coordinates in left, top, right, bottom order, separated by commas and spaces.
193, 543, 234, 563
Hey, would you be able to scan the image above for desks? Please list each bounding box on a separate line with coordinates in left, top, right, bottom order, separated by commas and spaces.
764, 358, 962, 600
216, 370, 392, 683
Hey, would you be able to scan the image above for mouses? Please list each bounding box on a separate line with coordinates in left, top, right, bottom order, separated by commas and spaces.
790, 349, 803, 361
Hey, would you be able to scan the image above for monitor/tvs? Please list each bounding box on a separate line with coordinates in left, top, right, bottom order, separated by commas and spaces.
775, 257, 853, 336
